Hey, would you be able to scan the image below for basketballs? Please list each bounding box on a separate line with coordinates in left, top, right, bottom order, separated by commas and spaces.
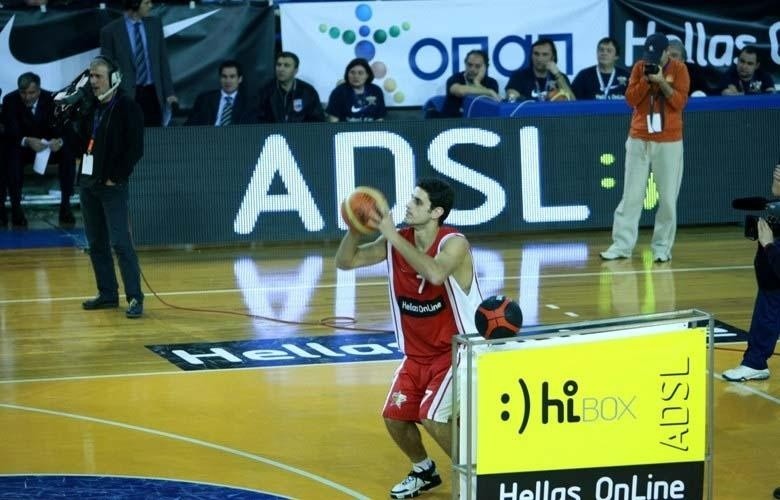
342, 187, 389, 237
476, 295, 523, 345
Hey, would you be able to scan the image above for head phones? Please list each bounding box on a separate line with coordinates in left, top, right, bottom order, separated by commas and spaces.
94, 54, 122, 87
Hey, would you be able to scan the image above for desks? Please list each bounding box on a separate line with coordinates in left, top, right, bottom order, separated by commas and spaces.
463, 94, 780, 119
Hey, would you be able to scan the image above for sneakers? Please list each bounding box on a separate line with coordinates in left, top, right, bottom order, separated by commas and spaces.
126, 298, 143, 318
599, 247, 632, 260
58, 200, 75, 224
654, 252, 672, 262
722, 365, 770, 382
11, 204, 29, 226
82, 299, 119, 310
390, 459, 442, 500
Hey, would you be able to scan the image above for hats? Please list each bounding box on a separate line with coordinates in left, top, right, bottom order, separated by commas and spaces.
641, 32, 669, 59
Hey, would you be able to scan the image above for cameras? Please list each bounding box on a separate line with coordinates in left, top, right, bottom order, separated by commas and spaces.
644, 64, 659, 84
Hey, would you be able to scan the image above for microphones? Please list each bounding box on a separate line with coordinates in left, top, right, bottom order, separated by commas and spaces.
97, 79, 121, 102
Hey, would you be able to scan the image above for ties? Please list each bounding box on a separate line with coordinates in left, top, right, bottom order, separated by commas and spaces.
219, 96, 233, 127
133, 21, 149, 85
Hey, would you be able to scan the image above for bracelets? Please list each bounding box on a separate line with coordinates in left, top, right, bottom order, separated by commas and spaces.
553, 72, 563, 80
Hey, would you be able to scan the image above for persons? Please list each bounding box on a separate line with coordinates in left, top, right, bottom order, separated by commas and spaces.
0, 71, 77, 230
504, 40, 576, 102
183, 59, 260, 126
256, 52, 324, 123
570, 37, 632, 100
323, 58, 388, 121
717, 44, 777, 95
597, 32, 692, 263
48, 55, 147, 320
440, 49, 503, 117
331, 176, 485, 500
721, 160, 779, 383
99, 0, 180, 125
668, 40, 708, 97
